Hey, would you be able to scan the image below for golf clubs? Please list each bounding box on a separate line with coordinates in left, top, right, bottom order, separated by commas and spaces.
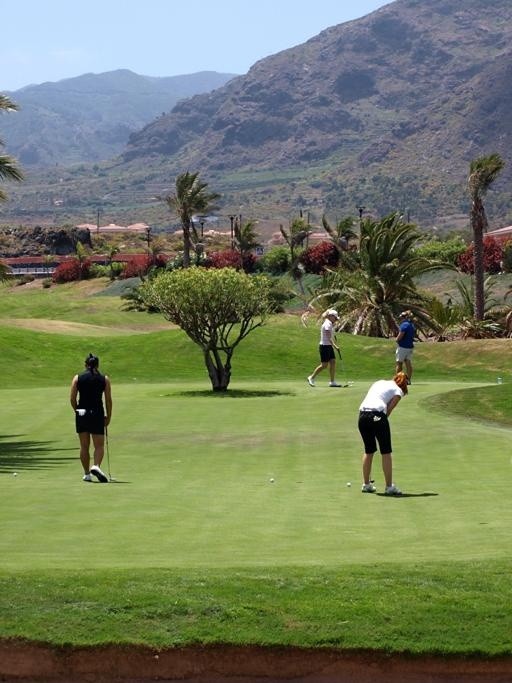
106, 426, 116, 481
337, 349, 349, 387
369, 476, 375, 484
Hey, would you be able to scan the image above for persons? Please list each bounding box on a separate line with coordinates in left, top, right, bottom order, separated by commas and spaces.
358, 371, 409, 496
70, 354, 112, 483
306, 309, 342, 387
395, 311, 416, 384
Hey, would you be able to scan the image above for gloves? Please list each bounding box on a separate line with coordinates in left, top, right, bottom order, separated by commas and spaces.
76, 409, 85, 416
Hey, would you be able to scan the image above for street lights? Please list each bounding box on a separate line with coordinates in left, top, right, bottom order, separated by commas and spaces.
197, 216, 209, 237
227, 215, 236, 249
355, 206, 366, 220
145, 228, 152, 256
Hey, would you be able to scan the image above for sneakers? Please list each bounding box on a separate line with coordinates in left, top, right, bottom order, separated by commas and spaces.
307, 376, 315, 387
409, 381, 411, 385
329, 382, 341, 387
385, 484, 401, 495
91, 465, 107, 482
362, 485, 376, 492
83, 475, 91, 480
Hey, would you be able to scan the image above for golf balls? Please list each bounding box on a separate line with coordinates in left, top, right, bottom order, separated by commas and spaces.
270, 479, 273, 481
14, 473, 17, 476
348, 482, 351, 486
348, 381, 354, 384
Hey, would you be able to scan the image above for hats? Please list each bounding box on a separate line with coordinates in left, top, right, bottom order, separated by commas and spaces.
329, 310, 340, 320
399, 312, 409, 317
